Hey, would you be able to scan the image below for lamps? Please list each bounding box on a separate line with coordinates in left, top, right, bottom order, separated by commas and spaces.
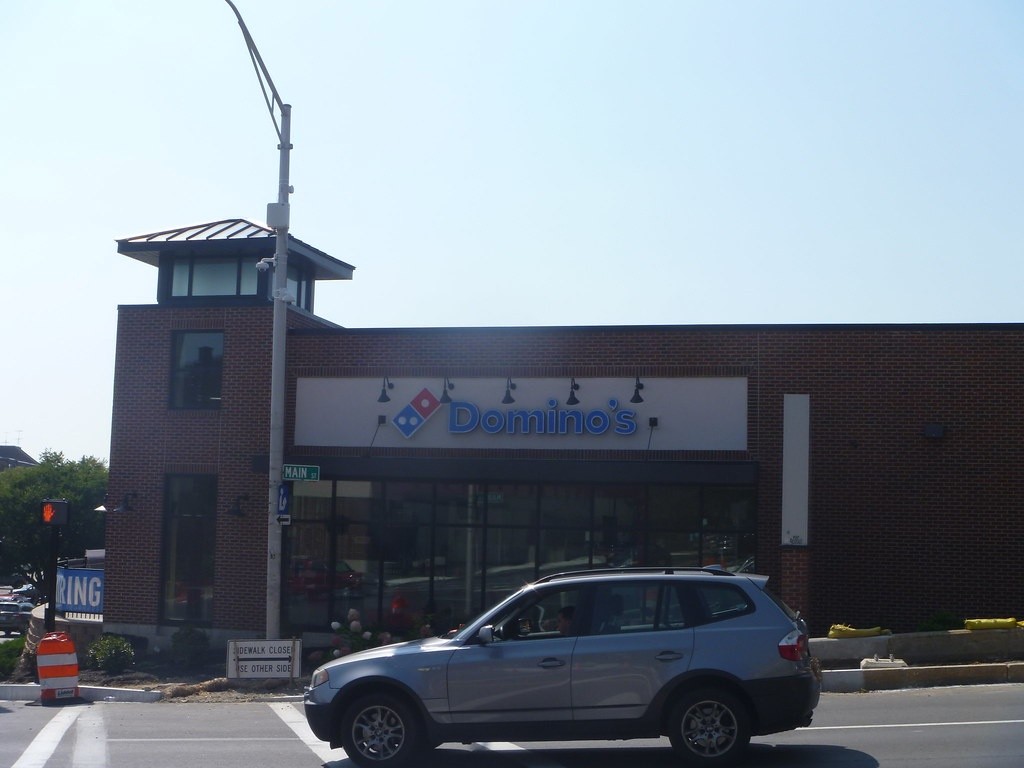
629, 375, 644, 403
566, 377, 580, 405
93, 493, 108, 512
502, 377, 516, 404
439, 377, 454, 403
924, 424, 944, 439
377, 376, 394, 402
112, 493, 136, 512
223, 495, 250, 517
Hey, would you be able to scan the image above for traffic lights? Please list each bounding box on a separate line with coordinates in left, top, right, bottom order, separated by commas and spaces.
39, 499, 71, 526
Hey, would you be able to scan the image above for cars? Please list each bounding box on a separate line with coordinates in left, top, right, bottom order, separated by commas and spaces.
0, 582, 49, 636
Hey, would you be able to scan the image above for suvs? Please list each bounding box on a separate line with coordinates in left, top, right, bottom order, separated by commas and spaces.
302, 563, 825, 768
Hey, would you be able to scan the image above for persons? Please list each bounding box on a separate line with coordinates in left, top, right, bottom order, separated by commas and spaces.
556, 607, 576, 638
331, 607, 369, 659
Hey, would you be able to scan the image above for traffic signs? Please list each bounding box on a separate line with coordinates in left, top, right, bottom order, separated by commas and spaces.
225, 639, 301, 679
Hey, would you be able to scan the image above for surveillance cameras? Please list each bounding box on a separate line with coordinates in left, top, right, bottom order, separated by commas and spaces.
256, 262, 270, 272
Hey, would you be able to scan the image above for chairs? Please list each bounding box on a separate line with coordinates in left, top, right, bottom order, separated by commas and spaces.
600, 594, 624, 633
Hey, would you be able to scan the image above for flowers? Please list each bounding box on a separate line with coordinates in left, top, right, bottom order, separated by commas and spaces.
307, 596, 434, 664
